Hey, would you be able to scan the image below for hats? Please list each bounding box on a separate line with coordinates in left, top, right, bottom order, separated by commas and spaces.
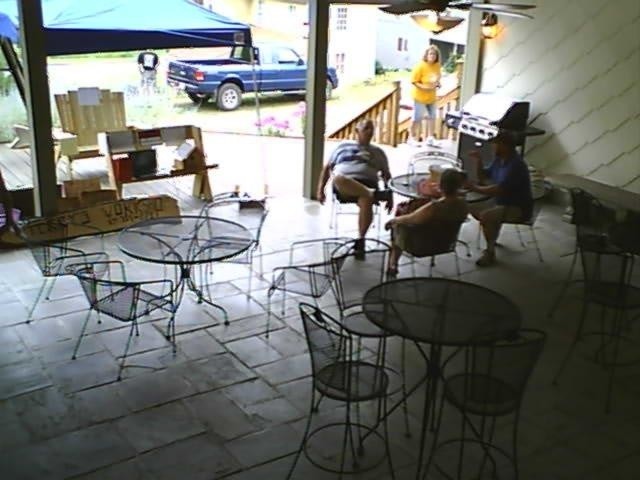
487, 133, 518, 145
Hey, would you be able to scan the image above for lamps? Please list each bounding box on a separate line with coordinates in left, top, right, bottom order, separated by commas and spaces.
480, 13, 498, 39
410, 11, 464, 35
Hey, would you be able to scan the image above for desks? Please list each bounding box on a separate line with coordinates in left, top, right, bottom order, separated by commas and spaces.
363, 277, 522, 479
116, 215, 253, 340
387, 173, 479, 267
608, 220, 640, 335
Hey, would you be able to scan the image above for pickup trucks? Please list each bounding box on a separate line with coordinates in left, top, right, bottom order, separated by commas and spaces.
168, 42, 338, 111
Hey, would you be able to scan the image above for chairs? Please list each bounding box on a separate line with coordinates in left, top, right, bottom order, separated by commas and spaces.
547, 187, 615, 319
265, 241, 349, 349
193, 191, 269, 304
312, 238, 411, 455
329, 169, 381, 244
477, 185, 551, 262
409, 151, 463, 173
391, 217, 471, 276
65, 261, 176, 381
553, 246, 640, 413
288, 302, 394, 479
424, 328, 546, 478
16, 223, 119, 324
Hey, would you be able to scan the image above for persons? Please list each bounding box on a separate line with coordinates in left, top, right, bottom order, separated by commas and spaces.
466, 133, 533, 269
410, 45, 442, 137
316, 118, 392, 260
386, 170, 468, 276
138, 50, 160, 91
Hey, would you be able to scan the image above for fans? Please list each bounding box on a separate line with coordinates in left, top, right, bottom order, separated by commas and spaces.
378, 0, 536, 21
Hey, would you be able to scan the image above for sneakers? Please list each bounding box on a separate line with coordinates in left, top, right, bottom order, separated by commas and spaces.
475, 249, 497, 267
384, 268, 400, 276
371, 188, 394, 201
351, 238, 367, 256
